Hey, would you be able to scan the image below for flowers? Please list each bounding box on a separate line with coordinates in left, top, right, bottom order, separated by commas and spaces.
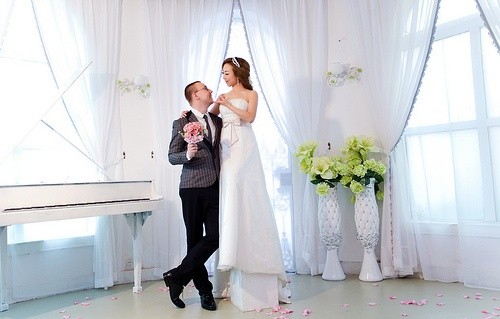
325, 61, 364, 89
336, 133, 387, 206
179, 121, 208, 144
294, 139, 349, 197
117, 73, 153, 100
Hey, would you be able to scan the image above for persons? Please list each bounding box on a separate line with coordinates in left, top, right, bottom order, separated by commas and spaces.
180, 56, 293, 304
161, 81, 223, 312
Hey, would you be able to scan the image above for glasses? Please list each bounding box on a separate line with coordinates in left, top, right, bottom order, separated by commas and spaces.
194, 86, 207, 94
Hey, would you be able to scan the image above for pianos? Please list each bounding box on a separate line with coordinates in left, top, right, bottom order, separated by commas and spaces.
0, 176, 167, 312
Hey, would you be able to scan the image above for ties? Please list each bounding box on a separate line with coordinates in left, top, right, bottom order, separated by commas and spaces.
203, 115, 213, 146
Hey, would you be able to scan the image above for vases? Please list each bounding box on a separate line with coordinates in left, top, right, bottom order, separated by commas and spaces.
354, 177, 383, 283
317, 180, 347, 281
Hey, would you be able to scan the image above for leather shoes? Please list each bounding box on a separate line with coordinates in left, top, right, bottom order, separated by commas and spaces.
163, 270, 185, 307
200, 289, 217, 311
